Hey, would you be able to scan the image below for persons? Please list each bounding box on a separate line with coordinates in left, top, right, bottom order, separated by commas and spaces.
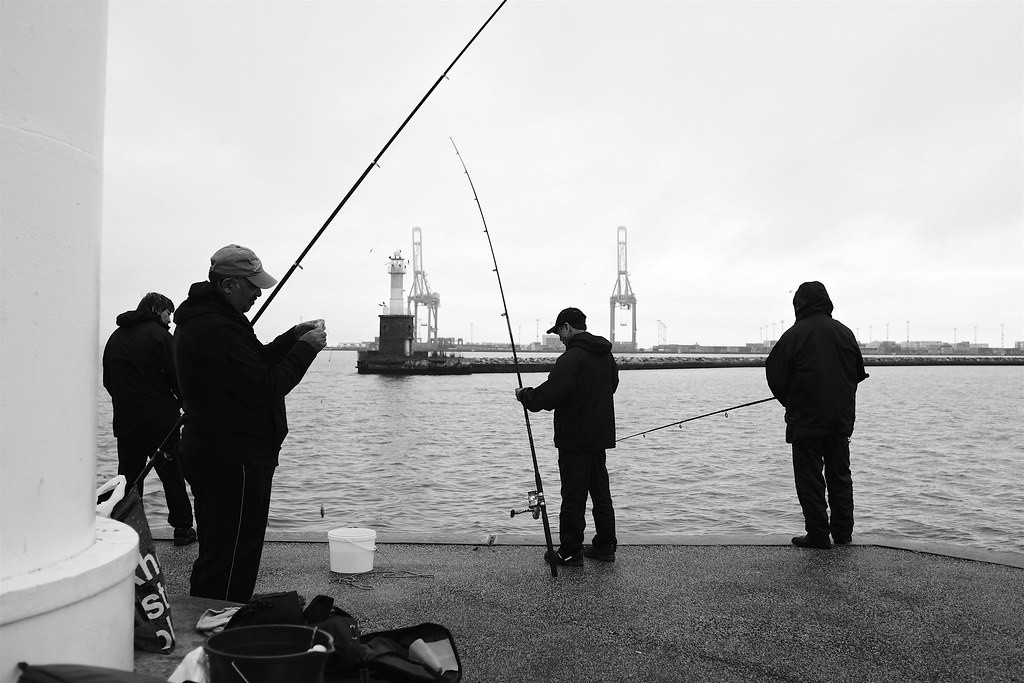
765, 280, 866, 548
103, 244, 327, 602
515, 308, 619, 566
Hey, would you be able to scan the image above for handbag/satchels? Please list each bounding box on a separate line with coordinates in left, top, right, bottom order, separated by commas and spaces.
220, 590, 462, 683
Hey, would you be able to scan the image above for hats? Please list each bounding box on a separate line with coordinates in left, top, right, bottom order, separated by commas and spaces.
546, 307, 587, 334
209, 243, 278, 289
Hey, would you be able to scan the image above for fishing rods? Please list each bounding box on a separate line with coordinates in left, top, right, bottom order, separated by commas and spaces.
446, 132, 559, 582
133, 0, 504, 489
614, 369, 871, 445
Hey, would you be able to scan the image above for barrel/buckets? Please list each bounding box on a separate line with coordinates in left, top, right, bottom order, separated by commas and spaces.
204, 623, 334, 683
327, 528, 377, 574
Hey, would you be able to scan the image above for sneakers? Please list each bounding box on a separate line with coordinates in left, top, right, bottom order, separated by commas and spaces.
544, 547, 584, 566
834, 535, 852, 545
583, 544, 615, 562
173, 527, 197, 546
791, 533, 832, 549
